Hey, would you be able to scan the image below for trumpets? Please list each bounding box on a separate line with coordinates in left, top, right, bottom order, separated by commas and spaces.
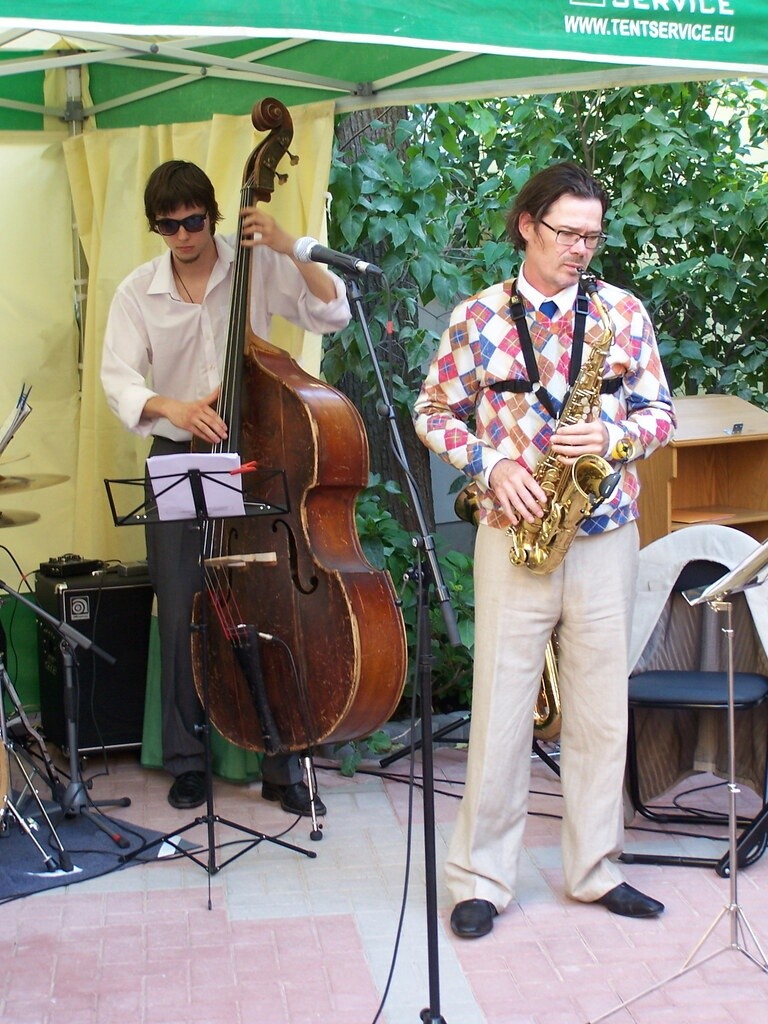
454, 480, 563, 742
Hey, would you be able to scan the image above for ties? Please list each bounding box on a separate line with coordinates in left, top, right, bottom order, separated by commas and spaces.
539, 301, 558, 320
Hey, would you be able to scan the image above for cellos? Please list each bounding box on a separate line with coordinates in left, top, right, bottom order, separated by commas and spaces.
186, 98, 409, 841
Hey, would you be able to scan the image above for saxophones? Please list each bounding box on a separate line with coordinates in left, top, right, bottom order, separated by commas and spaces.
506, 267, 619, 576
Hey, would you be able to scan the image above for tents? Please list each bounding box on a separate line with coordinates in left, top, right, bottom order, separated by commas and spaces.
0, 0, 768, 142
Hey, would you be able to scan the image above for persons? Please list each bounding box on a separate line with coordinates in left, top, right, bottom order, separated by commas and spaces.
98, 158, 353, 819
410, 160, 679, 942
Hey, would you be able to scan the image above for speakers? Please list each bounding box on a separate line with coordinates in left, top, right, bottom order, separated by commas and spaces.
35, 570, 158, 754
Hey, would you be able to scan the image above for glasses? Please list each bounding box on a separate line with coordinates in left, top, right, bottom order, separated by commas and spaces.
154, 209, 207, 236
540, 220, 607, 249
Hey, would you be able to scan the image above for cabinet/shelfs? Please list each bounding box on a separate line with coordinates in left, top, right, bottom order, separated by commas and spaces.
630, 393, 768, 548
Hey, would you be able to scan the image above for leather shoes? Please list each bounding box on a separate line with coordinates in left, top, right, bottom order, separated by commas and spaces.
262, 780, 326, 816
168, 771, 207, 809
593, 882, 664, 917
450, 899, 496, 938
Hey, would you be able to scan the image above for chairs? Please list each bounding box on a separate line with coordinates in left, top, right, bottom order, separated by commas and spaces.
621, 525, 768, 869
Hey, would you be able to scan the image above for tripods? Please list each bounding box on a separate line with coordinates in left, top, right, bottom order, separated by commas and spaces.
104, 465, 317, 909
0, 582, 133, 851
585, 538, 768, 1024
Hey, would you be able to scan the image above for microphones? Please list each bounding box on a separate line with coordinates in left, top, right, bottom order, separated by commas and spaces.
293, 237, 385, 277
